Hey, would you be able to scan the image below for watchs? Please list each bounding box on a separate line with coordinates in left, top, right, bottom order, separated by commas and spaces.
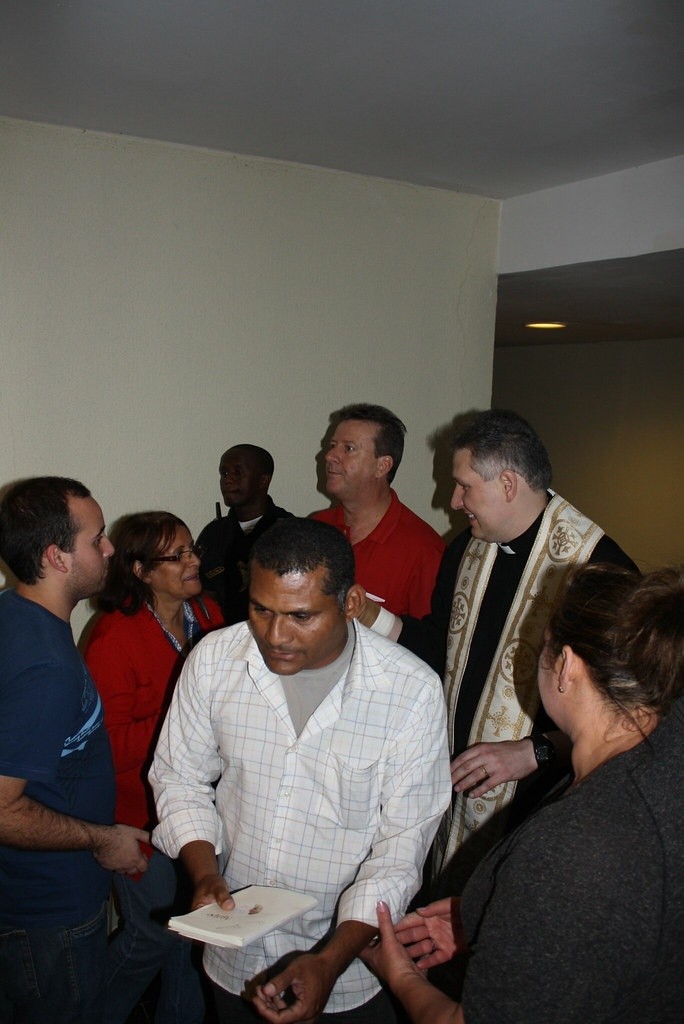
526, 734, 556, 771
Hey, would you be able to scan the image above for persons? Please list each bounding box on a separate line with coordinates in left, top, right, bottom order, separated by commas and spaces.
356, 411, 642, 1002
83, 511, 227, 1023
147, 516, 452, 1024
362, 565, 684, 1023
306, 403, 445, 619
0, 477, 149, 1024
195, 445, 296, 624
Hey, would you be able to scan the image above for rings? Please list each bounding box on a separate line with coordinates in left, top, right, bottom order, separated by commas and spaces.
430, 939, 436, 951
480, 766, 489, 778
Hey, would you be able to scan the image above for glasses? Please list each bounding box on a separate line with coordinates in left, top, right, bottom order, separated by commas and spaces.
149, 546, 202, 565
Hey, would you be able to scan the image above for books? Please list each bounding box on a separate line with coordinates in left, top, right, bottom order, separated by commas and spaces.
168, 885, 319, 947
